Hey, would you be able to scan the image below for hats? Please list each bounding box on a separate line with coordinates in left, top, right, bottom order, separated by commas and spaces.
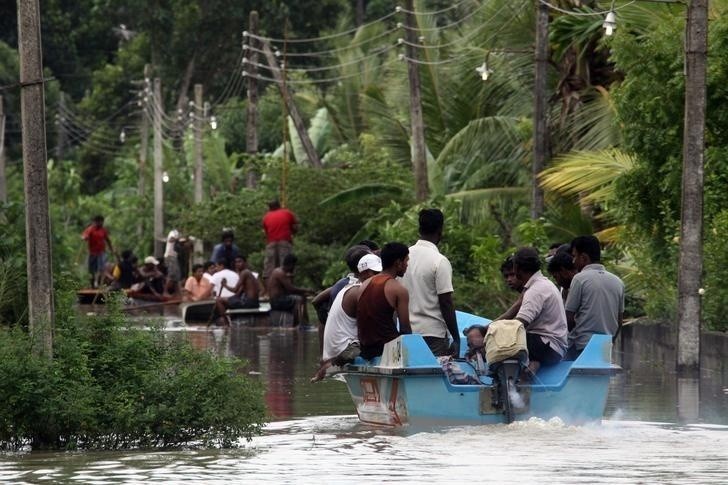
144, 256, 159, 265
357, 254, 382, 274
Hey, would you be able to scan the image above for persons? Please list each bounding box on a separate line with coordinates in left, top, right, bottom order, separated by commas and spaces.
312, 209, 461, 382
80, 198, 313, 334
465, 236, 625, 379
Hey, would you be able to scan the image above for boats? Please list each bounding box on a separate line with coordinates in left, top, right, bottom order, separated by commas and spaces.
329, 309, 623, 431
180, 299, 229, 325
226, 302, 302, 328
75, 287, 178, 305
